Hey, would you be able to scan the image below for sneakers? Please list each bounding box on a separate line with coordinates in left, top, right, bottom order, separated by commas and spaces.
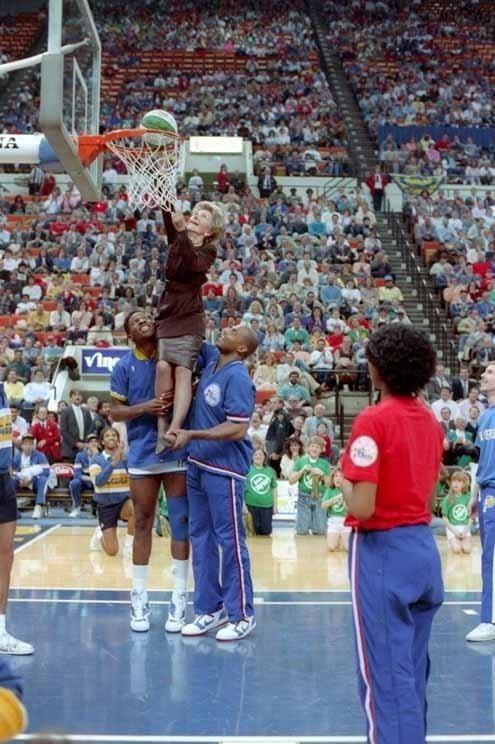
129, 593, 257, 641
89, 525, 102, 550
122, 534, 133, 546
31, 504, 44, 518
0, 631, 34, 655
68, 506, 81, 518
465, 622, 495, 641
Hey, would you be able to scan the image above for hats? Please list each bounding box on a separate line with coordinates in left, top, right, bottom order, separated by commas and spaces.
22, 433, 33, 440
87, 433, 98, 440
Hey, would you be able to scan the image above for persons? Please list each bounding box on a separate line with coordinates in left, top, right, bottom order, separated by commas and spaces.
109, 309, 201, 634
0, 1, 495, 557
166, 324, 259, 640
0, 652, 29, 743
465, 361, 495, 641
0, 381, 33, 654
339, 321, 446, 744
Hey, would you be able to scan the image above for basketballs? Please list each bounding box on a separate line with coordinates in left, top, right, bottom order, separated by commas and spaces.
139, 110, 178, 145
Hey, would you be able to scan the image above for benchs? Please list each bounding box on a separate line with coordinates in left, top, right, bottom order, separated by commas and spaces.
14, 485, 96, 520
322, 414, 359, 450
